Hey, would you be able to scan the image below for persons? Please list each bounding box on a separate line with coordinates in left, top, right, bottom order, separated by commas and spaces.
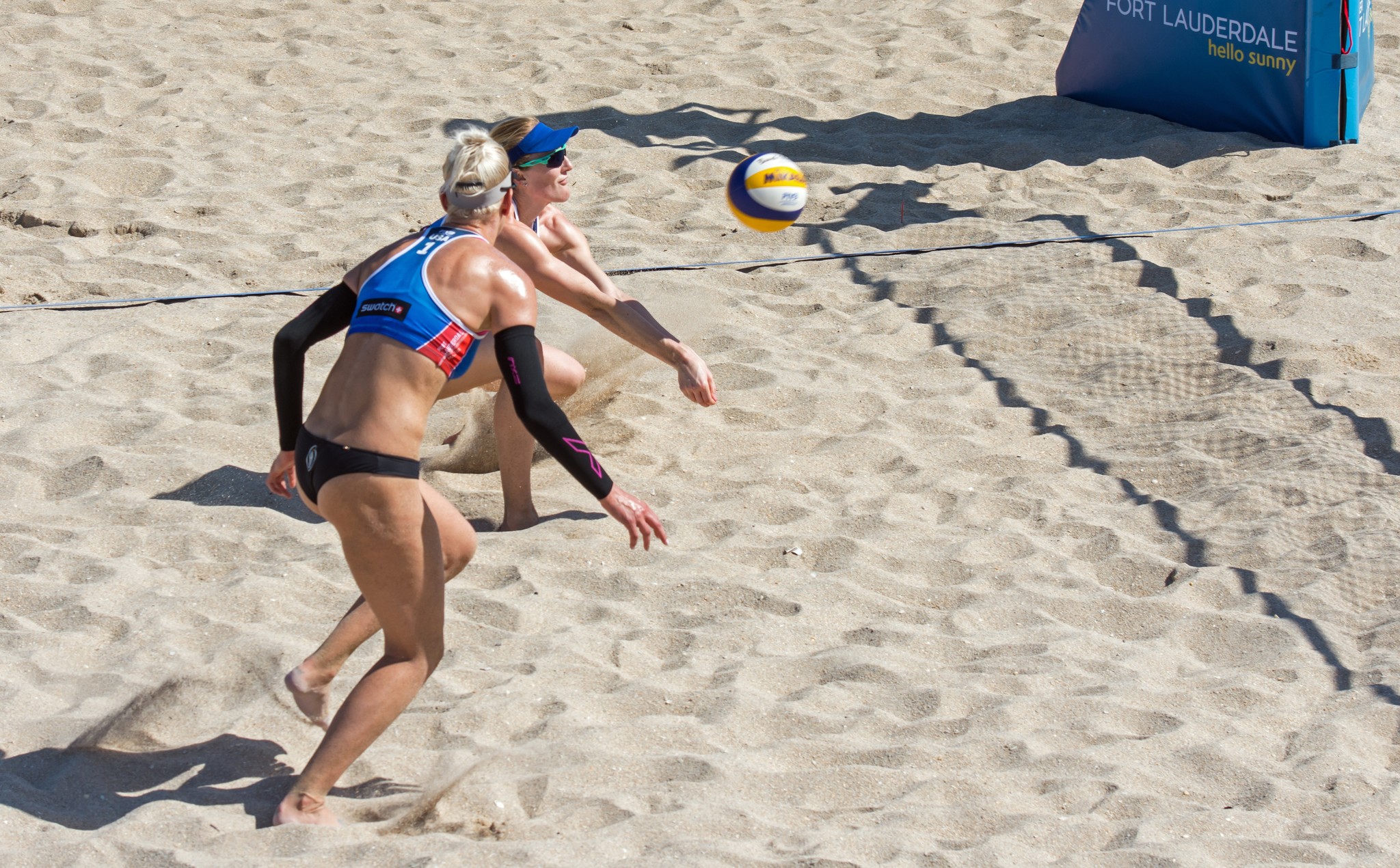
434, 115, 720, 534
264, 129, 671, 826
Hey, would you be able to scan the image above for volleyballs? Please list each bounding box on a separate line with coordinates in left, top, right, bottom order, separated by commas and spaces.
725, 151, 808, 233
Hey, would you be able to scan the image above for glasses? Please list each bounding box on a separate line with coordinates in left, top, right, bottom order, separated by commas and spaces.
515, 143, 568, 170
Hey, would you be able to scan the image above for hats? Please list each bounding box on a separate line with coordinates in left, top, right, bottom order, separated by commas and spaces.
507, 121, 580, 164
